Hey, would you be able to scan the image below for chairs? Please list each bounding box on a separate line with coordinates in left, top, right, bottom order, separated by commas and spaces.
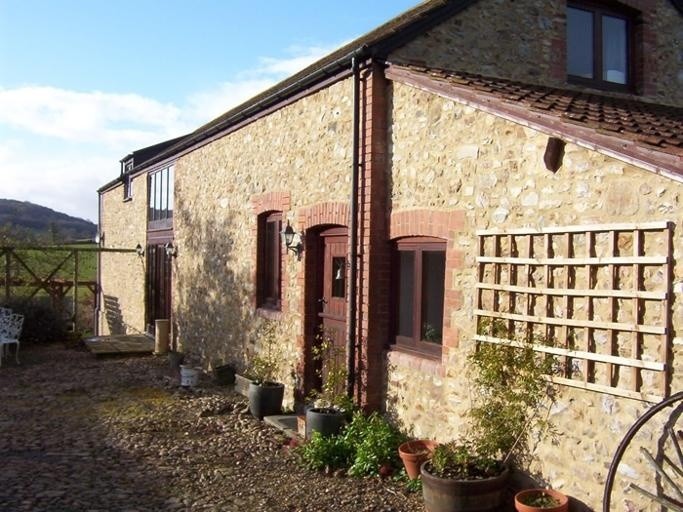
0, 308, 26, 367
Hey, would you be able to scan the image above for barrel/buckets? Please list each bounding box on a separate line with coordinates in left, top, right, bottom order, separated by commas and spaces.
180, 369, 198, 386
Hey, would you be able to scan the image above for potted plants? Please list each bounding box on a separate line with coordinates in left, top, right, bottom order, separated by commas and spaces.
248, 313, 354, 446
419, 307, 582, 512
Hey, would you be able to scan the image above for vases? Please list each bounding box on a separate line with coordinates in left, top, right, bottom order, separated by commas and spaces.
398, 439, 436, 483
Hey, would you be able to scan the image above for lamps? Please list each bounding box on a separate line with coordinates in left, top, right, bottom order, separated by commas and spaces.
166, 243, 177, 257
135, 243, 144, 257
279, 220, 301, 261
